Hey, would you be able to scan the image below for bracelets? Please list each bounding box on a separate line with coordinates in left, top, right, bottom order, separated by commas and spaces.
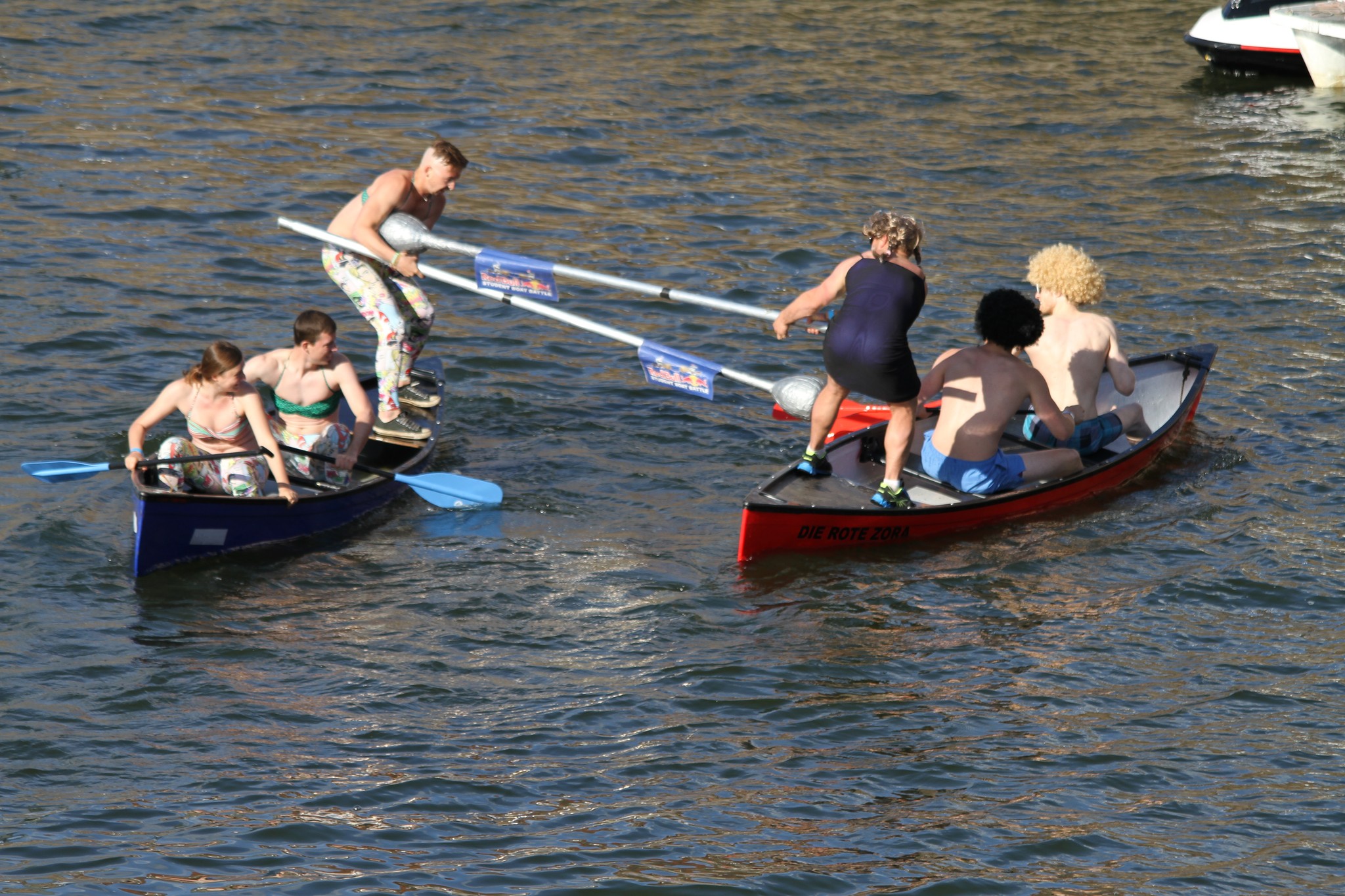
129, 448, 144, 456
391, 253, 400, 265
1062, 410, 1075, 421
828, 310, 835, 320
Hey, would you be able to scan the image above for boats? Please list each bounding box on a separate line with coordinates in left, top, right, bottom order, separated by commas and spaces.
129, 355, 443, 581
1182, 0, 1306, 77
736, 341, 1221, 562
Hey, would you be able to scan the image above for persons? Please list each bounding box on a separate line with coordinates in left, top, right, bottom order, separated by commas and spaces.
773, 210, 928, 510
916, 288, 1082, 493
238, 308, 374, 487
322, 140, 469, 440
124, 341, 299, 504
1011, 242, 1153, 455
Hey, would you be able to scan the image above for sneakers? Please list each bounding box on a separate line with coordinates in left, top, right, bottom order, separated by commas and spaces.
797, 448, 833, 477
371, 410, 432, 440
870, 481, 918, 509
397, 382, 441, 408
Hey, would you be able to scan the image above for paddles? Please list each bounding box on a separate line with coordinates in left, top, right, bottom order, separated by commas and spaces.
771, 386, 1038, 446
279, 445, 503, 509
19, 445, 274, 484
